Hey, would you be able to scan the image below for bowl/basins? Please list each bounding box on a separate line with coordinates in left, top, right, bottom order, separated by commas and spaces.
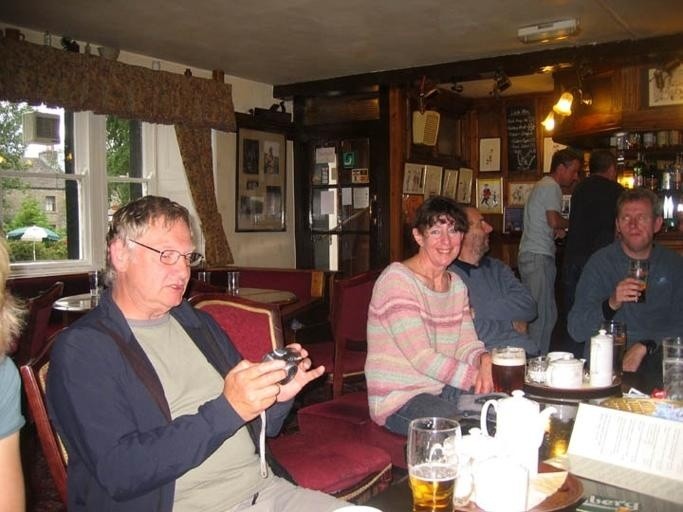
97, 46, 121, 61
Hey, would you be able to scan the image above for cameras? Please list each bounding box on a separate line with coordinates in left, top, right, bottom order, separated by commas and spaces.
260, 348, 302, 386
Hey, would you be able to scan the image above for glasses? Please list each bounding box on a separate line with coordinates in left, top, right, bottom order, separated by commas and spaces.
130, 239, 204, 267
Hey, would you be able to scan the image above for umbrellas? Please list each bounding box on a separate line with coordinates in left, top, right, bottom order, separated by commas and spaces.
6, 224, 60, 261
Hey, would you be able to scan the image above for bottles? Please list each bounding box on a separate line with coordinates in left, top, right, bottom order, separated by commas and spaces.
645, 153, 660, 192
672, 151, 683, 191
632, 152, 645, 193
659, 161, 674, 192
590, 328, 613, 387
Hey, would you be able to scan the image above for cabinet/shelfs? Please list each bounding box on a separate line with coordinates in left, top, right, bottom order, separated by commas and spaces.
612, 129, 683, 233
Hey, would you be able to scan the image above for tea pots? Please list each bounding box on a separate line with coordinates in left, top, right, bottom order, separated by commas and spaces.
544, 356, 587, 389
479, 390, 558, 449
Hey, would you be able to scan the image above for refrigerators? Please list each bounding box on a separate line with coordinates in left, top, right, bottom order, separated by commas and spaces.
308, 134, 387, 274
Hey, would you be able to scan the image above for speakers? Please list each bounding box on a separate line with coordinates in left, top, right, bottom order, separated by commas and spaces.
412, 109, 441, 147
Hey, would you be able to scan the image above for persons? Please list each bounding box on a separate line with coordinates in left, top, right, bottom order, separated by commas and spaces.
567, 189, 683, 396
450, 202, 539, 363
265, 146, 276, 173
44, 194, 353, 512
364, 193, 495, 447
481, 184, 490, 206
0, 235, 30, 512
518, 148, 580, 354
561, 150, 624, 356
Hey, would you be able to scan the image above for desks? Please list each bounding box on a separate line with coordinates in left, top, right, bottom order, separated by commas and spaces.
406, 368, 622, 511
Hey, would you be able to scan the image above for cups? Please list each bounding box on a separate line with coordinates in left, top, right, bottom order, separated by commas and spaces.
662, 334, 682, 397
406, 417, 461, 512
5, 27, 24, 41
197, 271, 211, 283
491, 346, 528, 394
151, 60, 160, 71
601, 319, 627, 380
226, 271, 239, 295
527, 356, 548, 383
87, 270, 104, 298
628, 257, 648, 305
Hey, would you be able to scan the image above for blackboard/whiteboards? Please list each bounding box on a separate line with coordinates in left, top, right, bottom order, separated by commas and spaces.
505, 103, 537, 173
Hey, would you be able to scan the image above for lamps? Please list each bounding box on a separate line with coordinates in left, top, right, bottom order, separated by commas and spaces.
540, 86, 592, 133
489, 69, 511, 106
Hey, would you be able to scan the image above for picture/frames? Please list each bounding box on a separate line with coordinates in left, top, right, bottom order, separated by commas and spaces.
640, 65, 683, 109
234, 128, 287, 233
403, 137, 538, 234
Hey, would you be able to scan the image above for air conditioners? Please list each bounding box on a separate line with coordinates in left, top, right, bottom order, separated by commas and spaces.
21, 109, 60, 145
